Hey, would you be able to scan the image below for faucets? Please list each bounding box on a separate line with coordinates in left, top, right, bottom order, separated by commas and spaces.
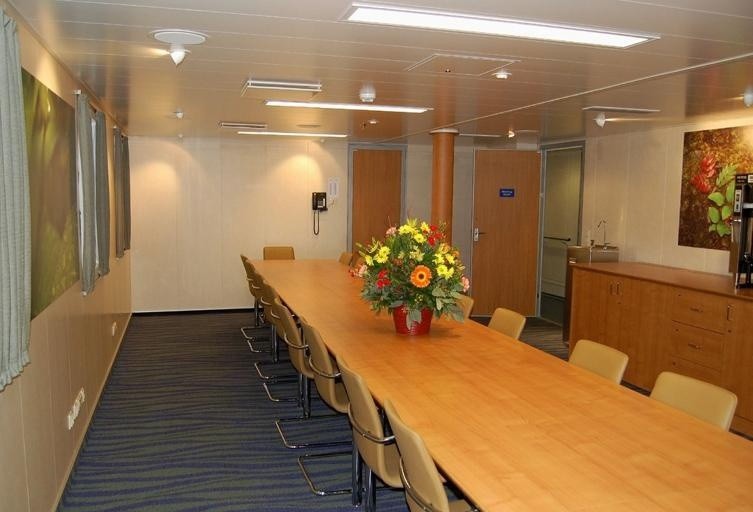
598, 220, 606, 244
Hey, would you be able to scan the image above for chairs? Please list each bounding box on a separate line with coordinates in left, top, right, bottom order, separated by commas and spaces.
240, 246, 738, 512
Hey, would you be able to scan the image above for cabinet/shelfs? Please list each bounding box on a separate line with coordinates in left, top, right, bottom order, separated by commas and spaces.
722, 297, 753, 437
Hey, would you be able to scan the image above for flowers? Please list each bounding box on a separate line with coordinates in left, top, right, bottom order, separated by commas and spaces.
346, 213, 469, 327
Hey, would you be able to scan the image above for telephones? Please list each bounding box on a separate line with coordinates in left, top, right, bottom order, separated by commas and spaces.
312, 192, 326, 210
